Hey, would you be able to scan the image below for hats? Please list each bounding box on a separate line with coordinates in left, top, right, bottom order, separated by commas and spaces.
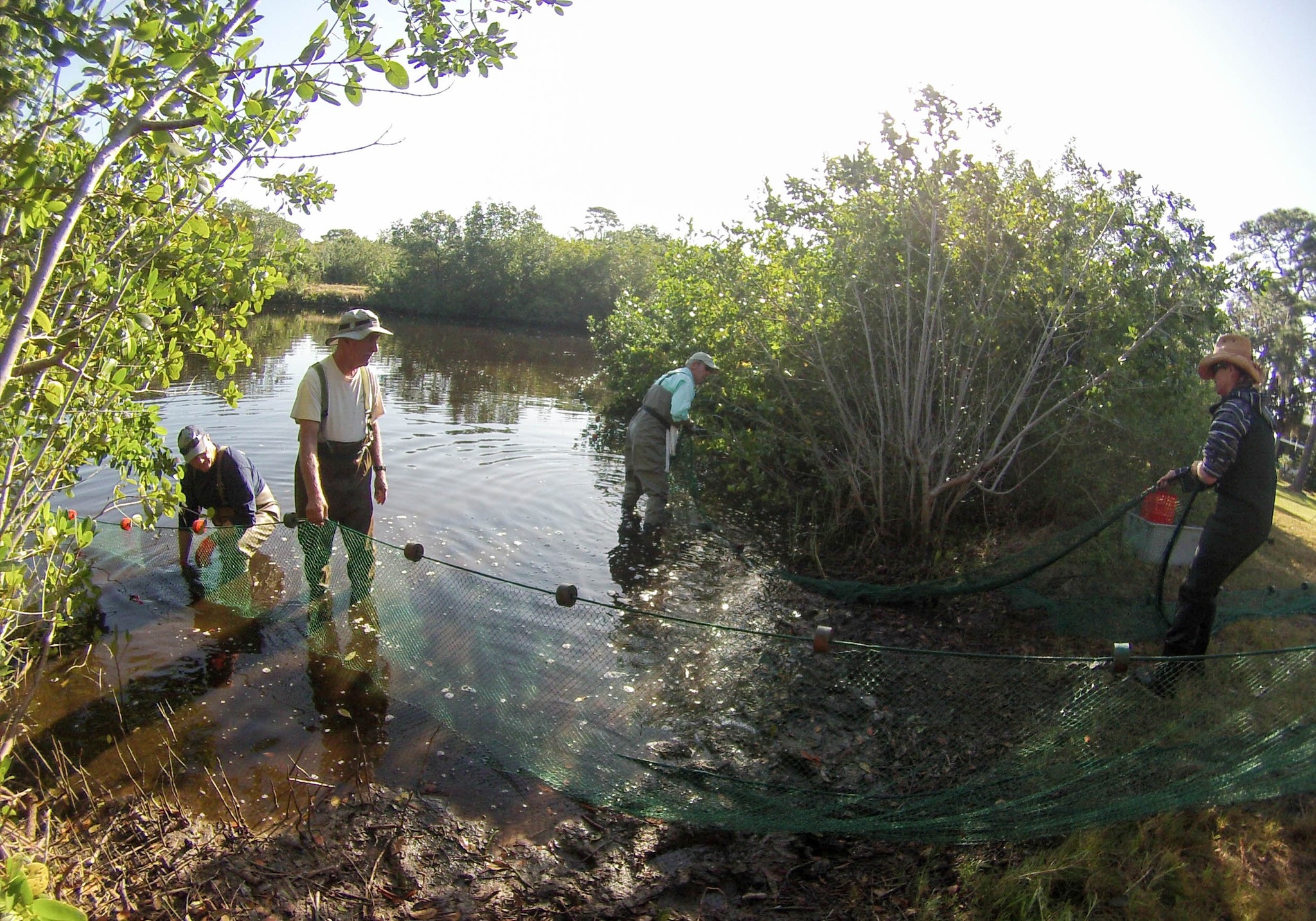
689, 352, 720, 370
325, 309, 394, 345
1199, 335, 1266, 382
178, 425, 208, 463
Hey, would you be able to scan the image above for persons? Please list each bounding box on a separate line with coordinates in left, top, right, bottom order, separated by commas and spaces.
1127, 334, 1277, 701
622, 352, 720, 524
290, 309, 393, 595
178, 424, 281, 582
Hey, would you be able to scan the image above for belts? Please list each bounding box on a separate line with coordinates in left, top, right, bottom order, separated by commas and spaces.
320, 441, 364, 454
643, 406, 671, 429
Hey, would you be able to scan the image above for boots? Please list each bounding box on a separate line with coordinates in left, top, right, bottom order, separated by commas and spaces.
1193, 629, 1211, 670
1132, 640, 1189, 698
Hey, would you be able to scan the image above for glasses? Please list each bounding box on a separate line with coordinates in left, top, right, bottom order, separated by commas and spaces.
699, 362, 711, 371
1211, 363, 1230, 373
180, 434, 203, 455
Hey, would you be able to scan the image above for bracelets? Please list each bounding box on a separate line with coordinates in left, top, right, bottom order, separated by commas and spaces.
373, 465, 386, 472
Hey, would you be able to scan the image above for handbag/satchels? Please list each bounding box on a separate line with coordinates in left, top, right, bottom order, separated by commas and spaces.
318, 438, 371, 490
211, 507, 238, 527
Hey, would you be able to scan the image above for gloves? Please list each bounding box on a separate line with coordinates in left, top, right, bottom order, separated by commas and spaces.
195, 536, 216, 567
